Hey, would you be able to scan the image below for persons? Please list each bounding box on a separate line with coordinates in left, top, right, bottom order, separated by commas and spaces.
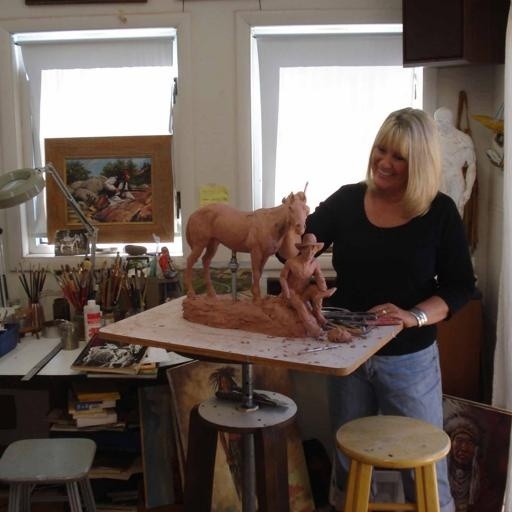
430, 108, 477, 220
101, 167, 137, 207
274, 107, 478, 511
278, 234, 329, 339
440, 414, 486, 511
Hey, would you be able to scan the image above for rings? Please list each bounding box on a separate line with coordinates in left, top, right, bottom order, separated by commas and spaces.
382, 309, 387, 314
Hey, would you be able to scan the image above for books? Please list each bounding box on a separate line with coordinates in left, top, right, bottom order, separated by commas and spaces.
42, 382, 142, 511
85, 364, 158, 381
136, 353, 157, 370
69, 333, 152, 375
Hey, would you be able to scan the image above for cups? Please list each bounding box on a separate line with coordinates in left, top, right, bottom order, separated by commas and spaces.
17, 316, 29, 328
59, 321, 80, 349
73, 314, 84, 341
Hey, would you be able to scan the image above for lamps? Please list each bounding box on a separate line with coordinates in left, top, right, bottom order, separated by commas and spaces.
0, 162, 100, 341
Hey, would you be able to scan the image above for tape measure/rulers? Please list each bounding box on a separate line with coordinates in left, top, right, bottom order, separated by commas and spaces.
21, 342, 64, 381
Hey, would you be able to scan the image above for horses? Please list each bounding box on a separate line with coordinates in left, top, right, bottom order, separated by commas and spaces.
183, 190, 310, 307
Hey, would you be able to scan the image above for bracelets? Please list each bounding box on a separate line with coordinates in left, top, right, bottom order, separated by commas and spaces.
408, 307, 429, 328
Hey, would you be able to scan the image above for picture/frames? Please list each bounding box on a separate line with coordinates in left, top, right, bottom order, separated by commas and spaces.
44, 135, 174, 245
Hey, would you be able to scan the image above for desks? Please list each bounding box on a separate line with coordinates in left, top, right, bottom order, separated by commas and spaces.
0, 332, 195, 512
98, 292, 404, 512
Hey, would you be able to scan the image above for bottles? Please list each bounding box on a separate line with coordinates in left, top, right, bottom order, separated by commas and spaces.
102, 307, 116, 325
53, 298, 71, 322
83, 300, 100, 340
28, 301, 45, 330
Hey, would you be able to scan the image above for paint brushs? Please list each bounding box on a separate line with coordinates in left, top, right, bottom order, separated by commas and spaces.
54, 262, 92, 314
17, 262, 49, 303
125, 265, 151, 311
93, 252, 125, 313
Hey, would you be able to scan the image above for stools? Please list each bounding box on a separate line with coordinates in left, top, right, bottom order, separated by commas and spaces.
0, 438, 97, 512
335, 416, 451, 512
